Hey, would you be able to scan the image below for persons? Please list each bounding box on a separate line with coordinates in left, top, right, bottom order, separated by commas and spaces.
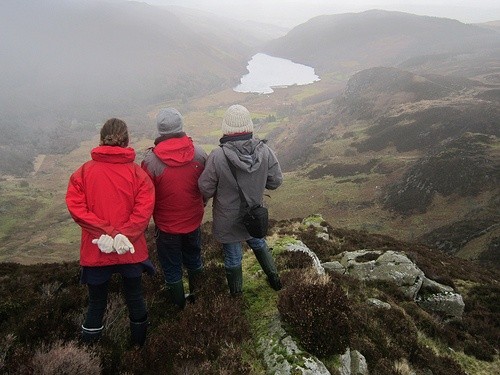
198, 105, 283, 295
140, 108, 210, 310
65, 118, 155, 349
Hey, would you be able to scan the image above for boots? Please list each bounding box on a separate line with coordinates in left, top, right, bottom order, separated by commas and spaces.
79, 325, 105, 350
186, 269, 203, 305
220, 265, 243, 306
129, 318, 148, 349
252, 247, 282, 292
165, 279, 186, 315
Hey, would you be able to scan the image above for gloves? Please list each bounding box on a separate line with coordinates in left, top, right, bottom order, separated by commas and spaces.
91, 233, 136, 255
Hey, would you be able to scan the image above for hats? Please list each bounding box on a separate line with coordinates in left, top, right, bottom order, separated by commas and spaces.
157, 108, 183, 134
221, 105, 253, 135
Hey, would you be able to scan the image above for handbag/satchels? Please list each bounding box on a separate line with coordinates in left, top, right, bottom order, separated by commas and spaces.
244, 204, 269, 239
